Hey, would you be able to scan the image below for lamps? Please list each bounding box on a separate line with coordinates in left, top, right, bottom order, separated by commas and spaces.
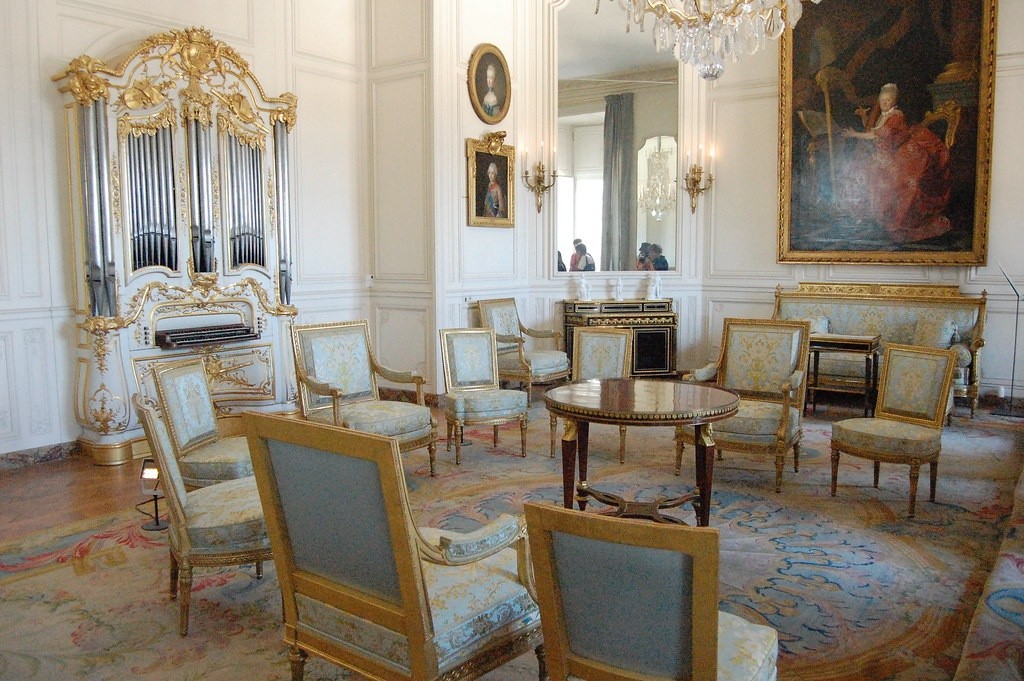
637, 134, 674, 221
685, 143, 721, 212
518, 143, 560, 213
595, 0, 801, 80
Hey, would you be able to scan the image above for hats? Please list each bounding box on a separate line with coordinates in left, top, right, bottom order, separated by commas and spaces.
640, 243, 651, 251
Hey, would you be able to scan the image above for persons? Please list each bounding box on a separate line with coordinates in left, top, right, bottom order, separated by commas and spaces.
558, 251, 567, 271
569, 238, 595, 271
636, 242, 668, 271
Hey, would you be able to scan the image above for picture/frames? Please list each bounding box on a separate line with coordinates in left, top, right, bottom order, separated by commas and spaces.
775, 0, 998, 265
465, 44, 512, 122
467, 132, 519, 229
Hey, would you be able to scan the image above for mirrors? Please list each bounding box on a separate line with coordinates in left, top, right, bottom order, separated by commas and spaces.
544, 325, 637, 464
550, 1, 703, 276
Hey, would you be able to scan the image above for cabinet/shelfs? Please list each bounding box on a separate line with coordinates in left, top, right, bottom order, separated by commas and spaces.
50, 25, 297, 467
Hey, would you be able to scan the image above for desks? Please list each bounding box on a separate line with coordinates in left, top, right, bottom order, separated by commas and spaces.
563, 299, 679, 379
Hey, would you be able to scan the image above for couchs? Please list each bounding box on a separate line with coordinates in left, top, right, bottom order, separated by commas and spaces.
755, 284, 985, 426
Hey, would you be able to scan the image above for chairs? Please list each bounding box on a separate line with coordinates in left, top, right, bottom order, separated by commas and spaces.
291, 317, 437, 483
243, 410, 543, 681
523, 502, 779, 681
833, 341, 955, 518
477, 297, 571, 408
670, 319, 810, 493
139, 396, 280, 639
437, 326, 529, 466
150, 356, 256, 513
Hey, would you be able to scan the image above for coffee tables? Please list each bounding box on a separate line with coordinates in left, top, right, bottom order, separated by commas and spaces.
801, 333, 883, 419
542, 382, 739, 525
